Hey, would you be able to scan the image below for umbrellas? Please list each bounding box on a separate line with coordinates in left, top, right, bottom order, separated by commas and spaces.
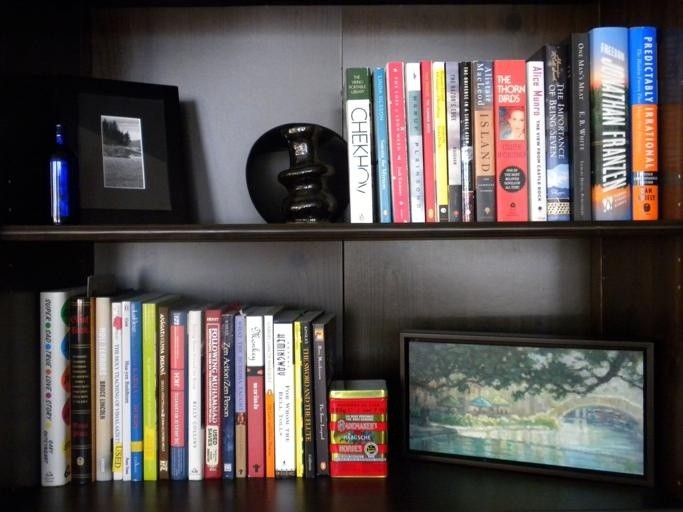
437, 393, 511, 416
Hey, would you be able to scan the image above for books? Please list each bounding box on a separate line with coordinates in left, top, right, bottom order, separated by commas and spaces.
40, 282, 337, 487
345, 27, 658, 222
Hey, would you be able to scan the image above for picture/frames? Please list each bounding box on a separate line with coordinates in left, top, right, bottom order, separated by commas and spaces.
396, 326, 658, 488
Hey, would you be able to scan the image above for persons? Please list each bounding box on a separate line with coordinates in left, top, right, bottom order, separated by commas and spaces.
500, 106, 525, 141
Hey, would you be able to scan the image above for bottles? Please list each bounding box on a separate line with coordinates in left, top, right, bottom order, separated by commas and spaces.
46, 122, 70, 227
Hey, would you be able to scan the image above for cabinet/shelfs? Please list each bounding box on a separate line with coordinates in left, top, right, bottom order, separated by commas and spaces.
0, 0, 683, 512
